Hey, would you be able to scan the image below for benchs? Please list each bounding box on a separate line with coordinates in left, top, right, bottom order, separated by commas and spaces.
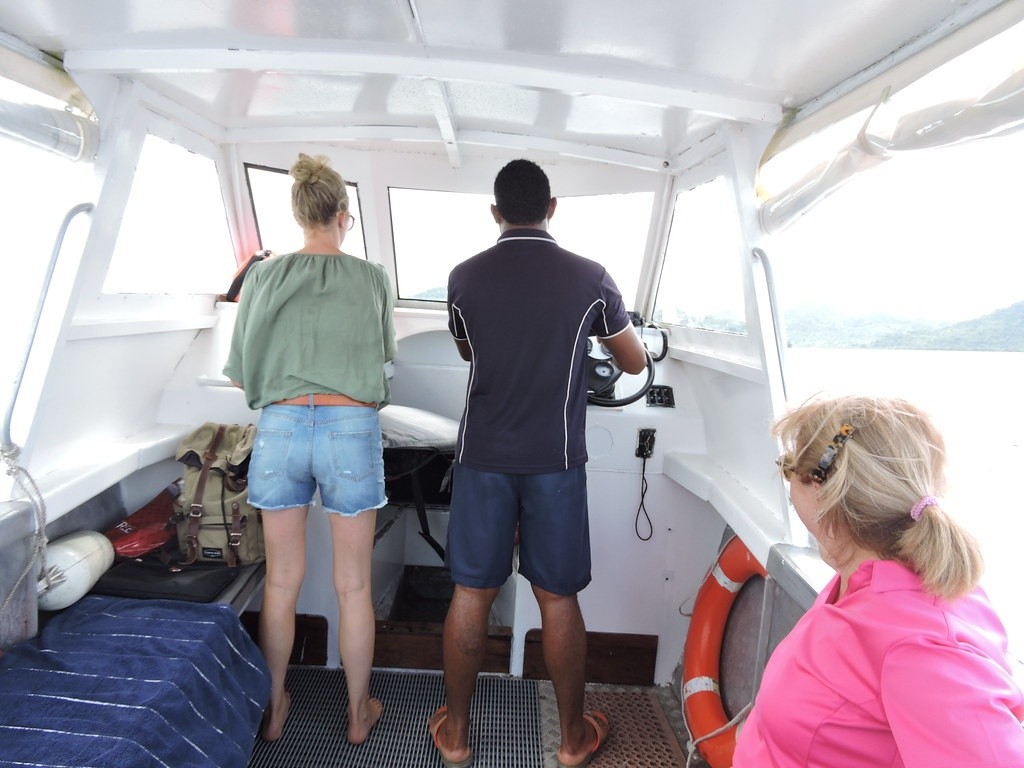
1, 558, 271, 768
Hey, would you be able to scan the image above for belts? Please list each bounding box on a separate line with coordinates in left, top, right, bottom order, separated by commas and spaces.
274, 393, 377, 409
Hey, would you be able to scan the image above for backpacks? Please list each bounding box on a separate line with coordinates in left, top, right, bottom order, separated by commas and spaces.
177, 421, 269, 568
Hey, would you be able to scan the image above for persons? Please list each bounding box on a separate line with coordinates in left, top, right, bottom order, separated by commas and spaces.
732, 397, 1024, 768
223, 153, 402, 743
427, 161, 647, 768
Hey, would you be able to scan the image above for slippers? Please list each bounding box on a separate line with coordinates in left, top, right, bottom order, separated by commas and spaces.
429, 708, 475, 768
557, 709, 610, 768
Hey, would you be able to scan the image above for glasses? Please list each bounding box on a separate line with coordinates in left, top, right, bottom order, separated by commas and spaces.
348, 214, 356, 231
776, 454, 796, 481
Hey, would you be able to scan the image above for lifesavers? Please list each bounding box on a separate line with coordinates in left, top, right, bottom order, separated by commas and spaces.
683, 535, 768, 768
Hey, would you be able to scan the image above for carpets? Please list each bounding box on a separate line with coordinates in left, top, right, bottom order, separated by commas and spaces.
246, 667, 545, 768
585, 690, 686, 768
